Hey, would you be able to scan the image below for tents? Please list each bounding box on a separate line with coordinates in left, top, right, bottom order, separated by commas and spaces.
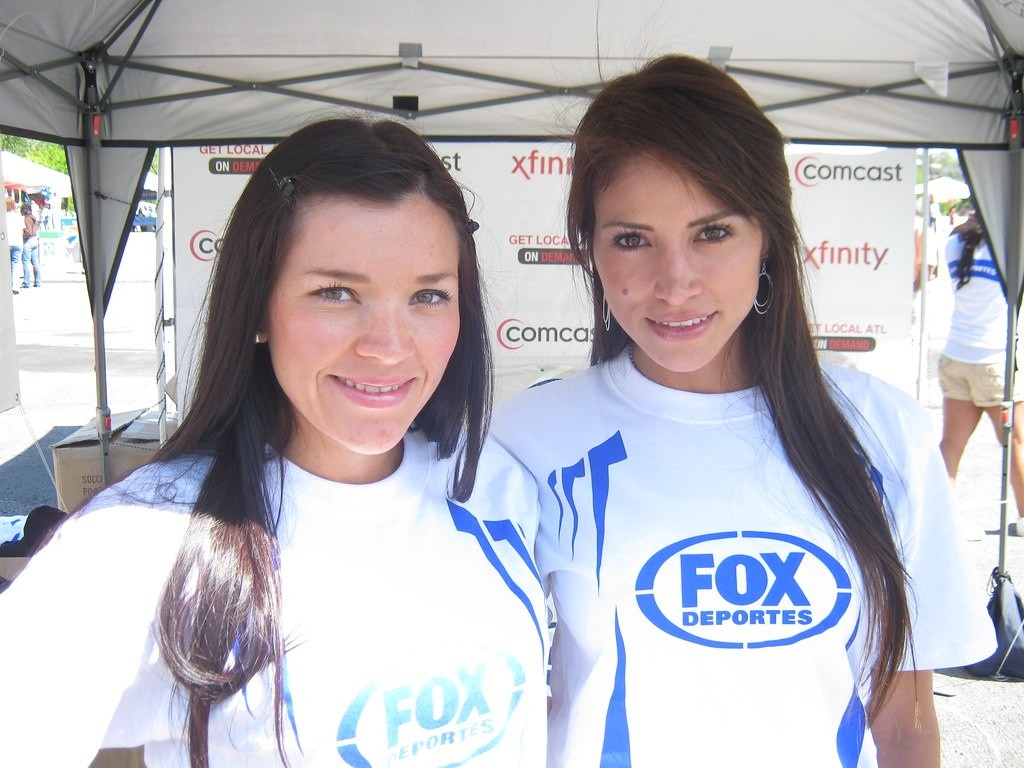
0, 148, 71, 202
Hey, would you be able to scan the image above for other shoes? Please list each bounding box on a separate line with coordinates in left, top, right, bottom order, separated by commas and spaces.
12, 290, 19, 294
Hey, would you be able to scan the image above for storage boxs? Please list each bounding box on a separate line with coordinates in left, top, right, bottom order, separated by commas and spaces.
48, 407, 177, 513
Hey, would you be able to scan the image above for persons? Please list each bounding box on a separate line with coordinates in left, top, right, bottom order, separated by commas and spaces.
938, 210, 1024, 535
19, 204, 41, 289
487, 56, 1000, 768
0, 118, 549, 768
6, 197, 26, 294
